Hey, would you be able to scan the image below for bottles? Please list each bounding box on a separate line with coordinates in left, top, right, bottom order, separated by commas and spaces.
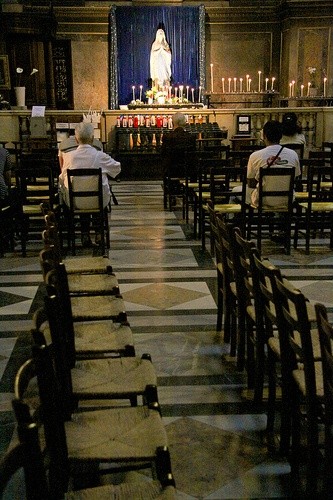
186, 114, 203, 139
160, 134, 163, 145
137, 134, 141, 146
129, 133, 133, 149
116, 114, 173, 129
152, 133, 156, 150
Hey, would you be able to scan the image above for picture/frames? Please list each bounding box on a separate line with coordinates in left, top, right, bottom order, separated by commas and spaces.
0, 55, 11, 90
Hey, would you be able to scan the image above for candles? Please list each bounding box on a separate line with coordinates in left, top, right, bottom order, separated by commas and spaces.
210, 63, 327, 97
132, 86, 135, 101
160, 85, 194, 103
199, 86, 201, 103
140, 85, 142, 101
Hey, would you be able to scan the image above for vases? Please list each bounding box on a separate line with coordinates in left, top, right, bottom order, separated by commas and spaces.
14, 87, 25, 107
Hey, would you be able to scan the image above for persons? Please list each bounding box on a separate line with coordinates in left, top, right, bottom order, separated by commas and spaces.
228, 120, 301, 242
280, 113, 305, 157
59, 123, 121, 245
162, 113, 197, 183
0, 148, 11, 204
59, 138, 103, 169
151, 29, 172, 85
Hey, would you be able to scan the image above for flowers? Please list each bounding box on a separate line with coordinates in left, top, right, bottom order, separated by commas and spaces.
15, 68, 38, 87
144, 86, 189, 104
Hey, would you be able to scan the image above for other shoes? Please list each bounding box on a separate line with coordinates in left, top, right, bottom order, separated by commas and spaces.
95, 234, 103, 245
81, 233, 92, 248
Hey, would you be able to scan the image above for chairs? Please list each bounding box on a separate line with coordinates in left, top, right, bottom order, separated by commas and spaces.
0, 138, 333, 500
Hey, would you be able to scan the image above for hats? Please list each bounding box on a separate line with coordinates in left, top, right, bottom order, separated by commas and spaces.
282, 112, 297, 122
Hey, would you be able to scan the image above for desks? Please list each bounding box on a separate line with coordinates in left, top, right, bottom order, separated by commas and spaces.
230, 139, 257, 151
